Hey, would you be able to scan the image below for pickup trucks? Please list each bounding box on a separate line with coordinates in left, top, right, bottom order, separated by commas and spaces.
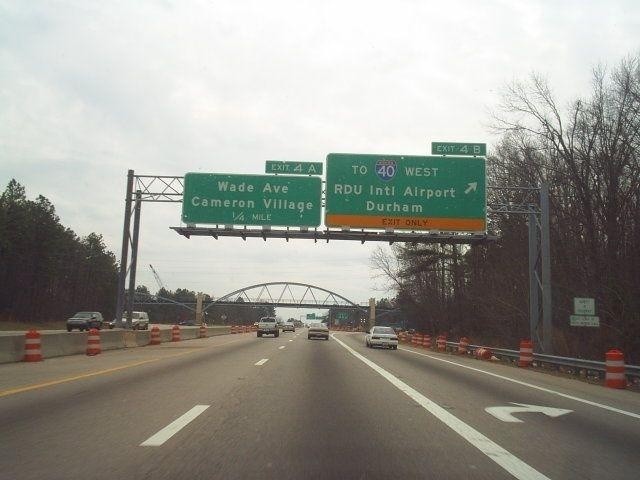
258, 317, 279, 337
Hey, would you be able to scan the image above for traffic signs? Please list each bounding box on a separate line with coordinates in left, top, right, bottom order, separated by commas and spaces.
324, 153, 488, 232
181, 171, 322, 228
335, 311, 346, 319
307, 314, 315, 319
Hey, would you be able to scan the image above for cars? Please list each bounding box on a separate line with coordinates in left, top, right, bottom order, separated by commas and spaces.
254, 322, 258, 325
364, 325, 398, 348
308, 323, 329, 340
282, 322, 295, 332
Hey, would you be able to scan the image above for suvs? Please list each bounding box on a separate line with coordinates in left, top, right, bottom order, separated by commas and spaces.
66, 311, 102, 331
110, 311, 150, 330
